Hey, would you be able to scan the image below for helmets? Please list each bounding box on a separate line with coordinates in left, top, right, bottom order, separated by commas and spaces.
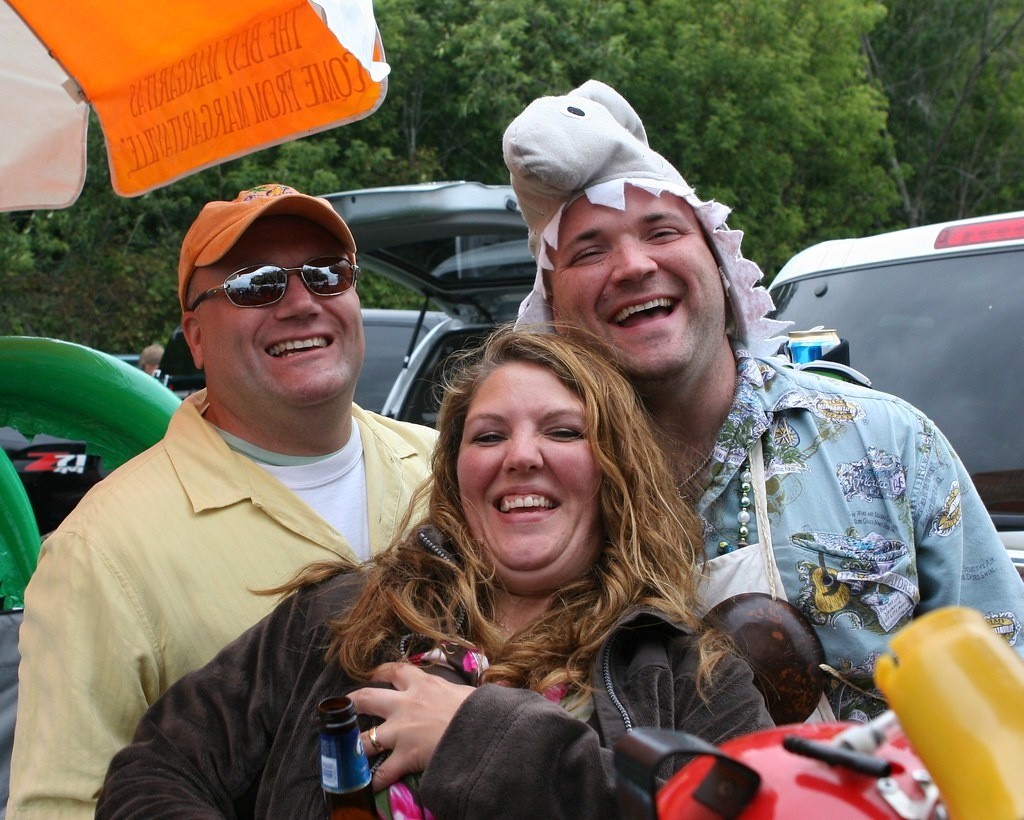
613, 723, 946, 820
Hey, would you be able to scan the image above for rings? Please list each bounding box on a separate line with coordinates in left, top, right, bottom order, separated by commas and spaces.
366, 725, 387, 753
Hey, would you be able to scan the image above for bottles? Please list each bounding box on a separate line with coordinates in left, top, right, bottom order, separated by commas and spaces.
317, 696, 383, 820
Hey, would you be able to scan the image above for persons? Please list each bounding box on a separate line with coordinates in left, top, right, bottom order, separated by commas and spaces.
5, 77, 1024, 820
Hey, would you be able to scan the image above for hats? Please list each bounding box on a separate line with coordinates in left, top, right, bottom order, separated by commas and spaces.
138, 344, 163, 369
177, 185, 357, 311
503, 76, 795, 372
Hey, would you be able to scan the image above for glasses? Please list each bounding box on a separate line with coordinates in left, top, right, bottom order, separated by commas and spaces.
190, 256, 360, 308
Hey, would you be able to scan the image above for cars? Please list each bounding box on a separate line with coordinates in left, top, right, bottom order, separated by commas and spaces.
0, 321, 209, 810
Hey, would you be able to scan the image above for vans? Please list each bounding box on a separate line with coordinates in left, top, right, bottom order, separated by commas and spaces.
316, 178, 540, 432
355, 307, 451, 414
763, 207, 1024, 577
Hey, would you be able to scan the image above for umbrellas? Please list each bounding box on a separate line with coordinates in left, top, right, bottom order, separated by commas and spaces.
0, 0, 391, 212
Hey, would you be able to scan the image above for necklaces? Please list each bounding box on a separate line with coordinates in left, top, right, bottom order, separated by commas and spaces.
488, 615, 516, 634
733, 458, 749, 553
672, 451, 715, 501
